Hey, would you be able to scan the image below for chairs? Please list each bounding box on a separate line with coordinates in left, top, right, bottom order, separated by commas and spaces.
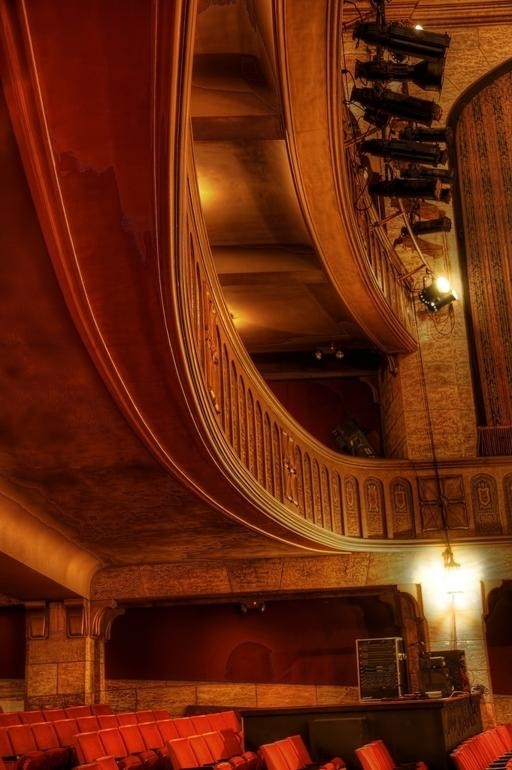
0, 704, 511, 770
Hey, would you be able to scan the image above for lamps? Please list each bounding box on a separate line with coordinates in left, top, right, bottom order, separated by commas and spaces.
350, 22, 453, 239
442, 547, 463, 583
419, 277, 458, 311
